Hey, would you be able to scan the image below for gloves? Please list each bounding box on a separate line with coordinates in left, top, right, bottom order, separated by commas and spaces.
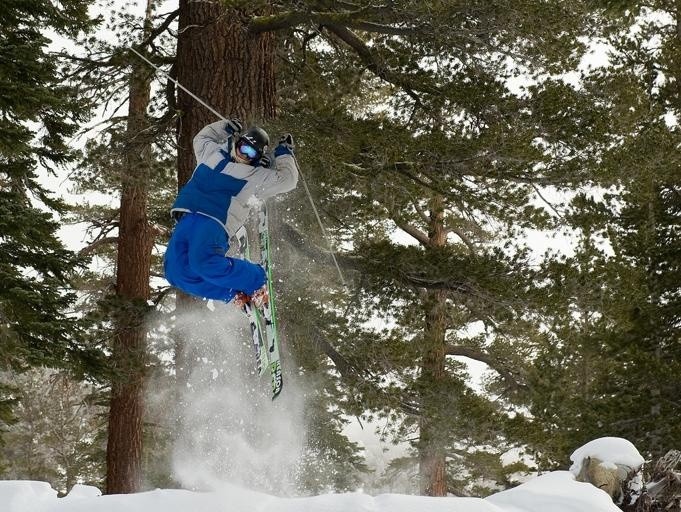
279, 133, 295, 151
226, 119, 242, 134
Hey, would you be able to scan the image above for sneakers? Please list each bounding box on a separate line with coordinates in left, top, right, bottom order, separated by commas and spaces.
251, 286, 267, 307
233, 293, 249, 307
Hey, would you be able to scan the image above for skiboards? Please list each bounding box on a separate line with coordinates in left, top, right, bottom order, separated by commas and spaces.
235, 198, 283, 401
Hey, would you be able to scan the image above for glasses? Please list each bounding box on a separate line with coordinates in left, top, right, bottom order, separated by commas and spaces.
239, 144, 257, 158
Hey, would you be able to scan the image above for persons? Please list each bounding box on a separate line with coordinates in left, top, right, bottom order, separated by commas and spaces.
164, 118, 300, 323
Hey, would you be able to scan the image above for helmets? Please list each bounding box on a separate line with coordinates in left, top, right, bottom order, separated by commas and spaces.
239, 126, 269, 154
